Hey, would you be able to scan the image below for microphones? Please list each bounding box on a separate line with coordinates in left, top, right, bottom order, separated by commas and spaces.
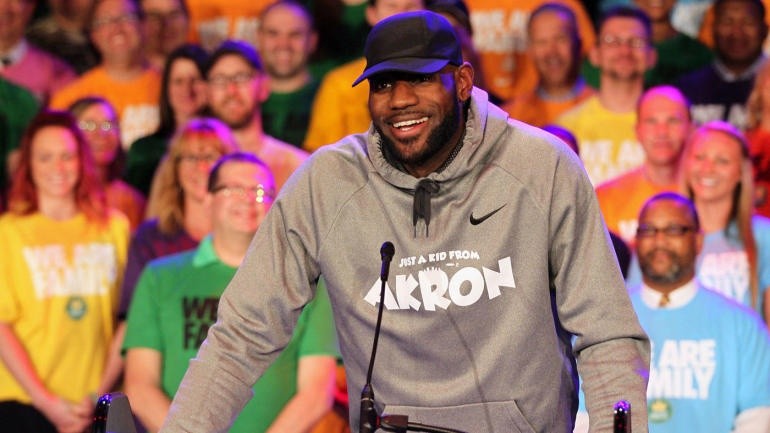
358, 241, 395, 433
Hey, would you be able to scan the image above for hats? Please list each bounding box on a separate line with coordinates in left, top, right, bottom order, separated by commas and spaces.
207, 39, 266, 73
352, 12, 464, 87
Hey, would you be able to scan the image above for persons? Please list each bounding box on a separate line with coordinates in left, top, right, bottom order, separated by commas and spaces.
0, 0, 770, 433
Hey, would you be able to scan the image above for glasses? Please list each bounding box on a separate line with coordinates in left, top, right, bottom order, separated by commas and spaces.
210, 70, 252, 84
636, 225, 690, 238
211, 183, 275, 203
93, 13, 134, 28
602, 35, 645, 48
82, 120, 114, 133
